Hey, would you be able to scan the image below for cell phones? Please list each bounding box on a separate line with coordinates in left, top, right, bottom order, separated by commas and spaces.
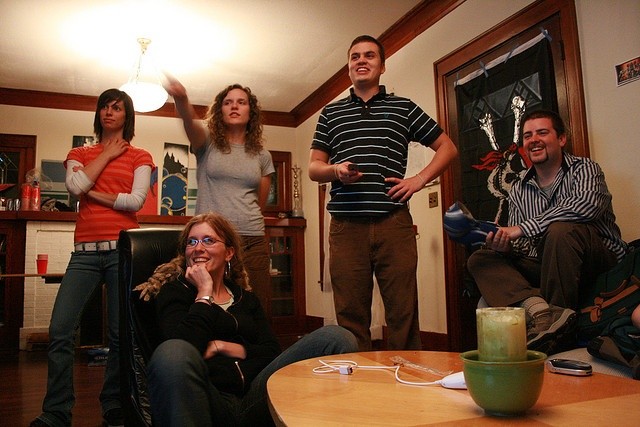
547, 359, 592, 376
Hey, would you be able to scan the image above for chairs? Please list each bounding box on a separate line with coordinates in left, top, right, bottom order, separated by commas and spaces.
117, 226, 266, 427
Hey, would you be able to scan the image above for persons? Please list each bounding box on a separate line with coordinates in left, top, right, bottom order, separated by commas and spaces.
29, 89, 153, 427
135, 216, 359, 427
163, 74, 274, 322
467, 110, 627, 354
308, 36, 459, 351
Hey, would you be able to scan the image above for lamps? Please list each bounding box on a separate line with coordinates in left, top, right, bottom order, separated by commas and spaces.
116, 36, 169, 115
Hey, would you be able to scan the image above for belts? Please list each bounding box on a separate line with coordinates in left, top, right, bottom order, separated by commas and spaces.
75, 241, 116, 252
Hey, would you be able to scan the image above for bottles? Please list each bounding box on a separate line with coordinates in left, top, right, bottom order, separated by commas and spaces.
6, 199, 13, 211
22, 183, 30, 211
13, 199, 21, 210
30, 181, 40, 211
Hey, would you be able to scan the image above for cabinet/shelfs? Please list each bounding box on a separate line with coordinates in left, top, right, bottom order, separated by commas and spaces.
22, 220, 82, 343
262, 226, 306, 332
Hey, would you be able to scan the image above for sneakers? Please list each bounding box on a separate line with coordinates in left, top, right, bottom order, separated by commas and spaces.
30, 409, 71, 427
526, 302, 575, 351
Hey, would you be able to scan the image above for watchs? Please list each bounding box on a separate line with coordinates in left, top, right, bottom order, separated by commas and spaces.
196, 296, 214, 303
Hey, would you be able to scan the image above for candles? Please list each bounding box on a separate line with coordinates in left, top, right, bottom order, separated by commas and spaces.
476, 306, 526, 361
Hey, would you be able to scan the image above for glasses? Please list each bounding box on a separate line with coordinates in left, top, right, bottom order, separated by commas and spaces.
184, 237, 228, 246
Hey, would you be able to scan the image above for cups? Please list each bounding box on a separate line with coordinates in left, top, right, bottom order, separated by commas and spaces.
37, 253, 48, 274
459, 349, 548, 418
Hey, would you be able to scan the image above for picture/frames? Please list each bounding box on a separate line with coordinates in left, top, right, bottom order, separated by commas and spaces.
613, 55, 640, 87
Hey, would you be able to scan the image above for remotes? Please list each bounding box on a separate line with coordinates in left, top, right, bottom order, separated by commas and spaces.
347, 163, 358, 173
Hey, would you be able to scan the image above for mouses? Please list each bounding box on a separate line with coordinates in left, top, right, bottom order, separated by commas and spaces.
441, 371, 467, 390
